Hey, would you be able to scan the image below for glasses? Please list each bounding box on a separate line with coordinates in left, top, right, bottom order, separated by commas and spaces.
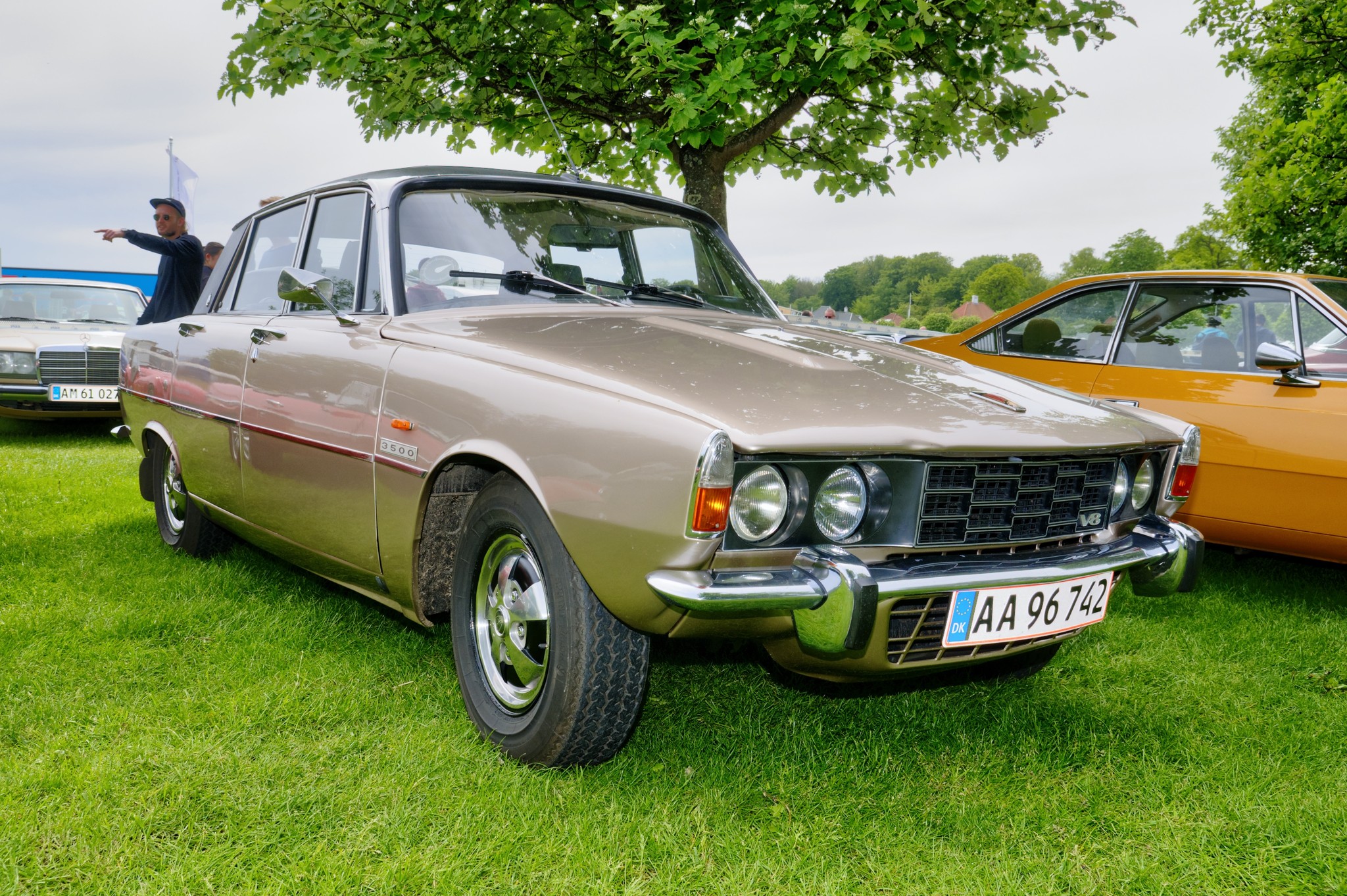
153, 214, 181, 221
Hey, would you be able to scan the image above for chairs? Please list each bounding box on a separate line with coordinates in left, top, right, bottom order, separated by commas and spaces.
337, 240, 406, 310
1136, 336, 1185, 367
542, 261, 583, 288
1202, 337, 1240, 369
1, 301, 35, 319
89, 303, 118, 320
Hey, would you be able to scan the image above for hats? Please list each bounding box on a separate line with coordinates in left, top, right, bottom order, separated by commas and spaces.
150, 198, 185, 218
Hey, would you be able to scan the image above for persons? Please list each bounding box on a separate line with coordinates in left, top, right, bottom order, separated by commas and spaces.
93, 198, 205, 326
200, 241, 225, 295
1191, 314, 1228, 351
1234, 313, 1276, 353
920, 326, 927, 330
800, 310, 815, 318
406, 257, 447, 306
258, 195, 322, 274
824, 308, 836, 319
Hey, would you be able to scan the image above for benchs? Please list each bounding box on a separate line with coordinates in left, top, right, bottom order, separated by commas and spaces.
1022, 318, 1135, 367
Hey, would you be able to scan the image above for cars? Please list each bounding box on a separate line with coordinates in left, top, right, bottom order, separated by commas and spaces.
108, 166, 1207, 775
0, 277, 149, 423
853, 269, 1347, 566
1292, 328, 1347, 375
